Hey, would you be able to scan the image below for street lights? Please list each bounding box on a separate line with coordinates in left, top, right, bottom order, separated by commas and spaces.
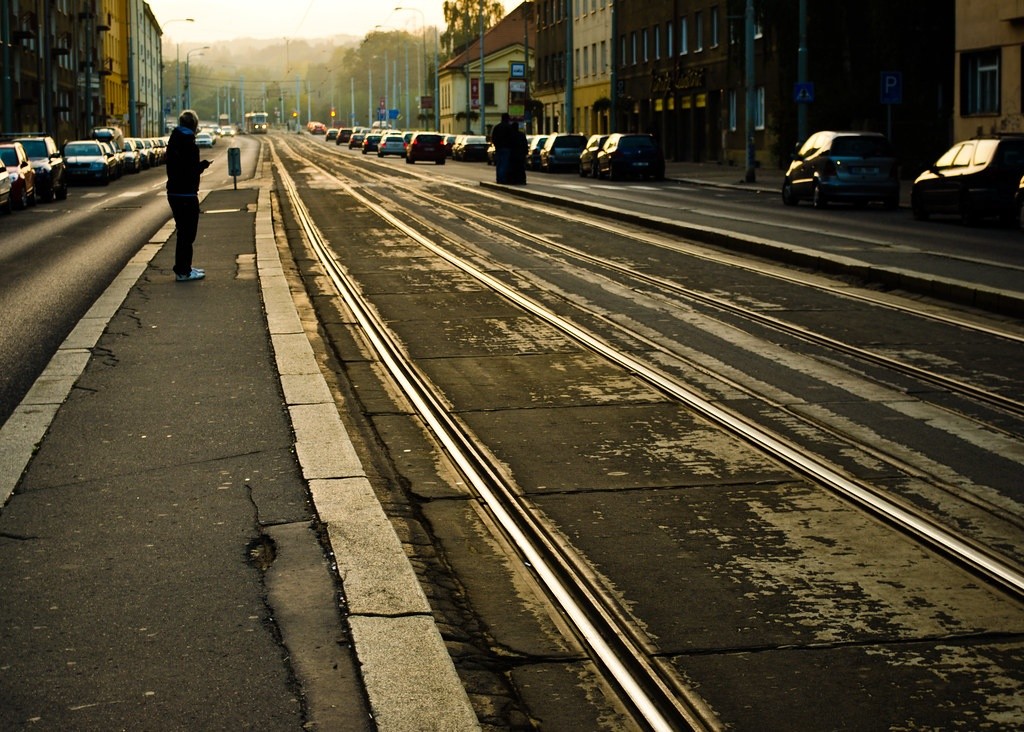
373, 25, 399, 130
395, 6, 429, 130
186, 45, 210, 111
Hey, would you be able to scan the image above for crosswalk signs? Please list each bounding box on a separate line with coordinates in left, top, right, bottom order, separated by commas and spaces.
794, 81, 815, 105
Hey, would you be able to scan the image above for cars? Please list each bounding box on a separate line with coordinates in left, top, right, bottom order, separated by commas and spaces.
361, 133, 382, 154
594, 131, 667, 182
325, 129, 338, 142
486, 143, 497, 165
781, 130, 904, 207
93, 127, 170, 180
306, 120, 326, 135
909, 131, 1024, 221
526, 134, 549, 171
194, 123, 236, 149
580, 134, 609, 179
348, 134, 365, 150
444, 133, 490, 163
372, 120, 393, 130
377, 135, 407, 158
0, 158, 13, 214
353, 126, 413, 143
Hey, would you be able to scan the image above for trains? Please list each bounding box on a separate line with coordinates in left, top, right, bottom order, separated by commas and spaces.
244, 110, 270, 134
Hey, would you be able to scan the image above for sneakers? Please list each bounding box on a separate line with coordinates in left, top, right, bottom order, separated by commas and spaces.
189, 267, 205, 273
173, 270, 206, 281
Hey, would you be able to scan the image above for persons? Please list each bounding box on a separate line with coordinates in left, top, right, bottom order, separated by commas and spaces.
164, 109, 211, 282
491, 112, 528, 184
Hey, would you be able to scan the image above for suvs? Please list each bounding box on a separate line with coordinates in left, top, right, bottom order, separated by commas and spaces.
62, 139, 112, 185
335, 128, 353, 145
404, 131, 446, 165
12, 136, 70, 202
538, 131, 588, 173
0, 142, 39, 209
